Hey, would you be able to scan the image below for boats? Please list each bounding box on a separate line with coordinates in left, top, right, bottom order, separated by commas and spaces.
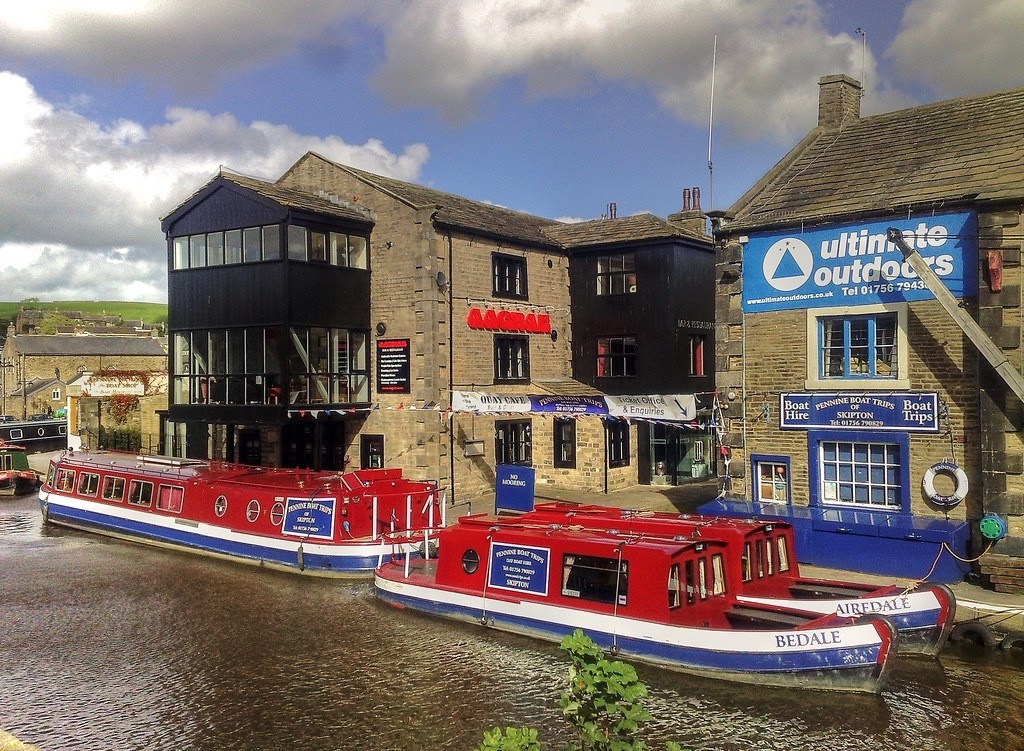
374, 513, 896, 695
528, 501, 959, 662
39, 446, 446, 580
0, 443, 37, 496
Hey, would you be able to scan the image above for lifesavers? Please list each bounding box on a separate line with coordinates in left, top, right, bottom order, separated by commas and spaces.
922, 462, 970, 507
